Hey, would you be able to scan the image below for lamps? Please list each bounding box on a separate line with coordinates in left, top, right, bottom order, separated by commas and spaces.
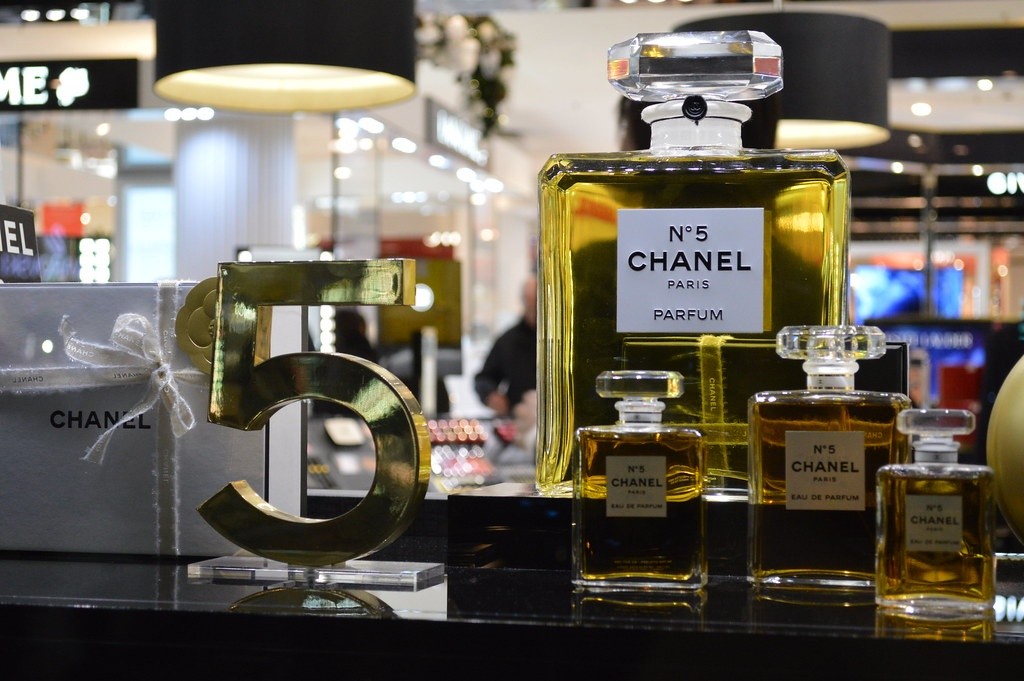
619, 1, 893, 152
152, 1, 416, 113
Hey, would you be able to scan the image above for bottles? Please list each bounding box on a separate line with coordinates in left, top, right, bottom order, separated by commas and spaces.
572, 371, 707, 594
888, 329, 985, 453
876, 410, 995, 622
748, 325, 911, 606
537, 29, 850, 494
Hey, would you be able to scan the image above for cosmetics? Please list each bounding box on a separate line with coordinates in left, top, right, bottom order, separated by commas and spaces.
537, 29, 852, 505
570, 328, 997, 616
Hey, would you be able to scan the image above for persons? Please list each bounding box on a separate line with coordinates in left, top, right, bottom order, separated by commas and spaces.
476, 278, 538, 419
571, 91, 823, 478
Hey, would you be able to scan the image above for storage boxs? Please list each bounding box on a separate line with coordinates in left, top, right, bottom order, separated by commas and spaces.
0, 284, 269, 557
